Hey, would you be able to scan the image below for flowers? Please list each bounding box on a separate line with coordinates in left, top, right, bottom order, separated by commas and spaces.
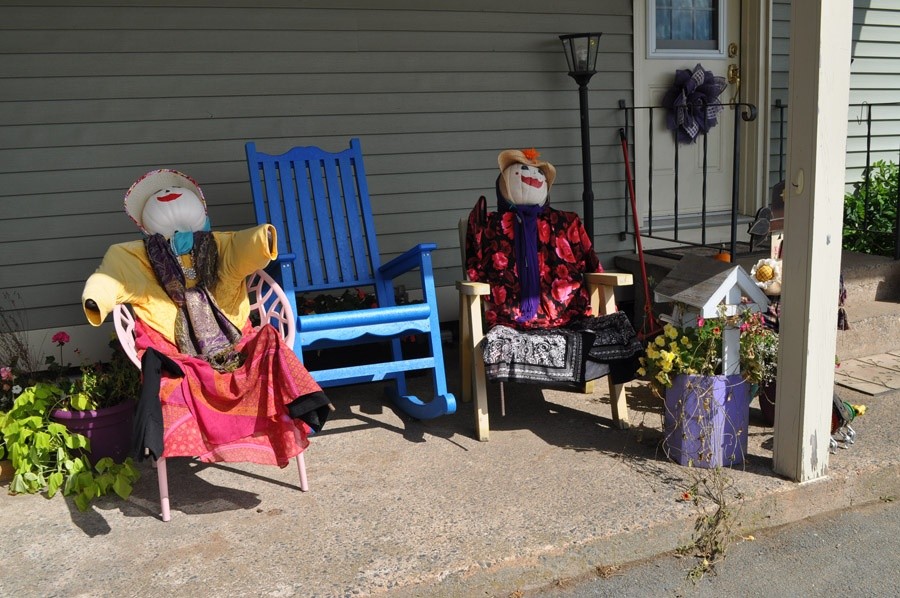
637, 306, 779, 390
0, 330, 141, 512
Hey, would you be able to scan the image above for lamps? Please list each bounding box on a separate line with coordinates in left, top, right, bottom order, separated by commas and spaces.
559, 31, 602, 85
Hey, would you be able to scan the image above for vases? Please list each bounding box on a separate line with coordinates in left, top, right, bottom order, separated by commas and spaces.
663, 374, 750, 467
52, 401, 135, 463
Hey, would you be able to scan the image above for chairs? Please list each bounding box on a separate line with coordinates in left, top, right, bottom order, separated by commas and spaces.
455, 217, 634, 443
113, 268, 309, 522
245, 137, 457, 436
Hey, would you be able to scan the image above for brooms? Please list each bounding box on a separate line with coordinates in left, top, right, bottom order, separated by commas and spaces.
616, 126, 666, 349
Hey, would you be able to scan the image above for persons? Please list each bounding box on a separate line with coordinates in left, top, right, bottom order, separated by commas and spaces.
465, 150, 645, 394
82, 170, 329, 469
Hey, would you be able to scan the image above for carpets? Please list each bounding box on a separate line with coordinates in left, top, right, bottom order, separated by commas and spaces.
644, 242, 771, 262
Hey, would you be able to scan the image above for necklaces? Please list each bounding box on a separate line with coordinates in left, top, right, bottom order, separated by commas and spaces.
169, 233, 197, 280
